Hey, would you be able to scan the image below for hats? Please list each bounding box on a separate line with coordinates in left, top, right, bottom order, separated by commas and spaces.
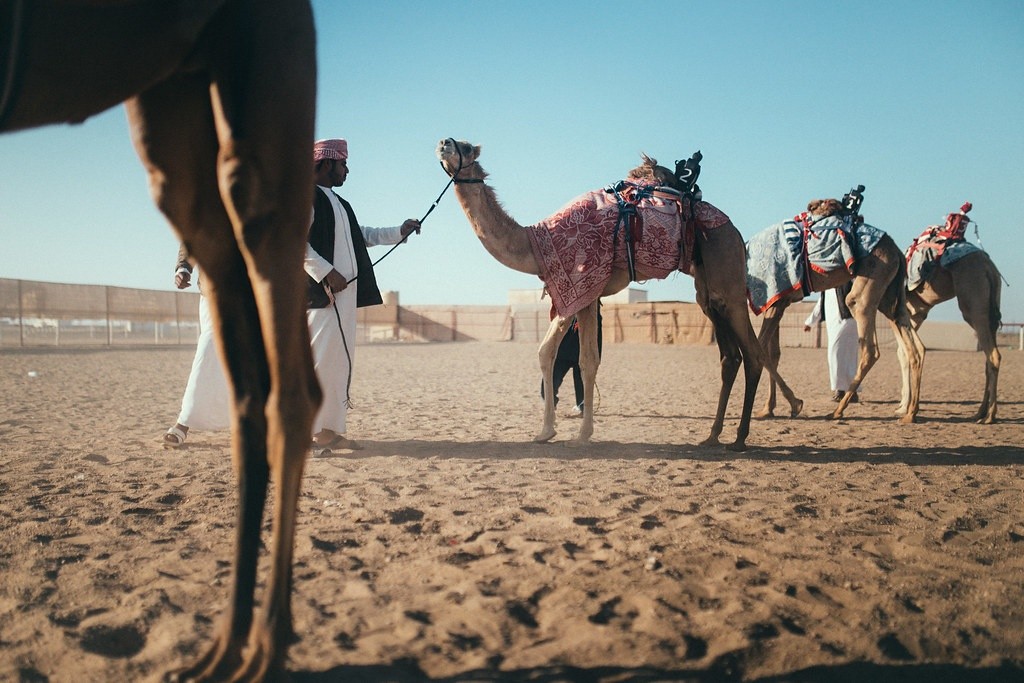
312, 138, 350, 162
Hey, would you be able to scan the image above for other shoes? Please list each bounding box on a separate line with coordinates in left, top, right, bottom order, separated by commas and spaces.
566, 406, 584, 418
832, 390, 858, 404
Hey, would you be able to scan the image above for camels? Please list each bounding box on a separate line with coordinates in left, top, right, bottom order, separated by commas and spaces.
2, 0, 319, 683
436, 138, 1009, 454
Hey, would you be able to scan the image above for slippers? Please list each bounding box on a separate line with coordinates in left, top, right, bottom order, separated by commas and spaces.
162, 428, 187, 447
310, 436, 366, 451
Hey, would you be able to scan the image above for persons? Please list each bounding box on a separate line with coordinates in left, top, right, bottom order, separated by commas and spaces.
160, 242, 234, 446
804, 279, 861, 403
302, 139, 420, 450
841, 185, 865, 217
541, 297, 602, 418
931, 202, 972, 251
669, 151, 702, 193
